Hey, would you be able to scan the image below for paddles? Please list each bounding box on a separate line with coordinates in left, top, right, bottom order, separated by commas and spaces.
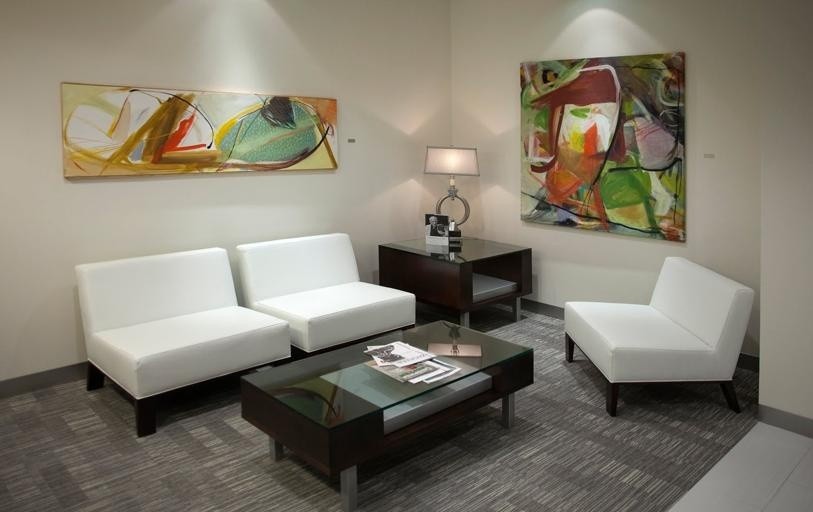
0, 302, 759, 512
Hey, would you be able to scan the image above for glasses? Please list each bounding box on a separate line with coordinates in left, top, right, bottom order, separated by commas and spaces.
422, 147, 481, 253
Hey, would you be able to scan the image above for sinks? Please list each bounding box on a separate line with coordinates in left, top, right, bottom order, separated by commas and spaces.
75, 247, 292, 437
239, 233, 416, 354
563, 257, 753, 416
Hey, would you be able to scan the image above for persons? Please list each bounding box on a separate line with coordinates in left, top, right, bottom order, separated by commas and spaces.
425, 216, 446, 237
